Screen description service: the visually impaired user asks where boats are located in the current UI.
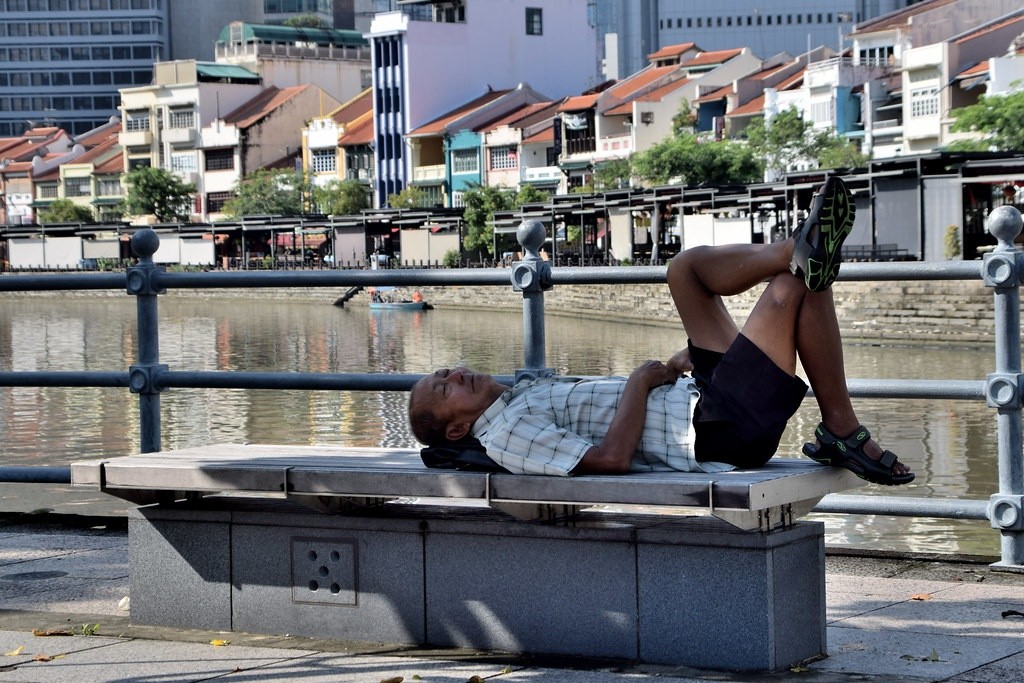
[368,299,427,310]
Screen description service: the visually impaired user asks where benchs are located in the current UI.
[71,443,868,675]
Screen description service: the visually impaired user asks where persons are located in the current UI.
[408,174,916,486]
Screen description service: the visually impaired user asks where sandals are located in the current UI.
[802,422,914,485]
[789,176,857,293]
